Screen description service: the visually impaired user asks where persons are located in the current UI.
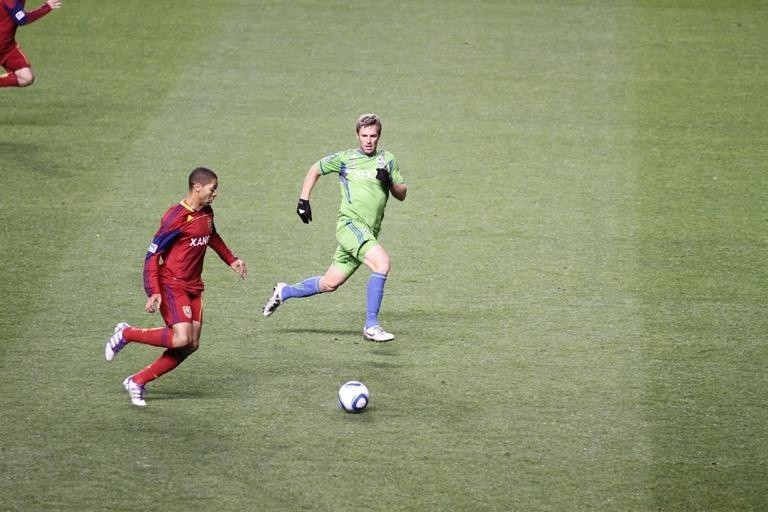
[1,0,62,87]
[262,114,408,342]
[104,167,248,408]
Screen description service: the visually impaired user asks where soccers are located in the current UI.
[339,382,369,413]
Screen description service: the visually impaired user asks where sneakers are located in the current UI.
[362,325,394,343]
[261,282,287,318]
[122,375,147,408]
[103,322,131,362]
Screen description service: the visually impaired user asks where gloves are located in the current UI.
[296,198,312,224]
[376,168,392,188]
